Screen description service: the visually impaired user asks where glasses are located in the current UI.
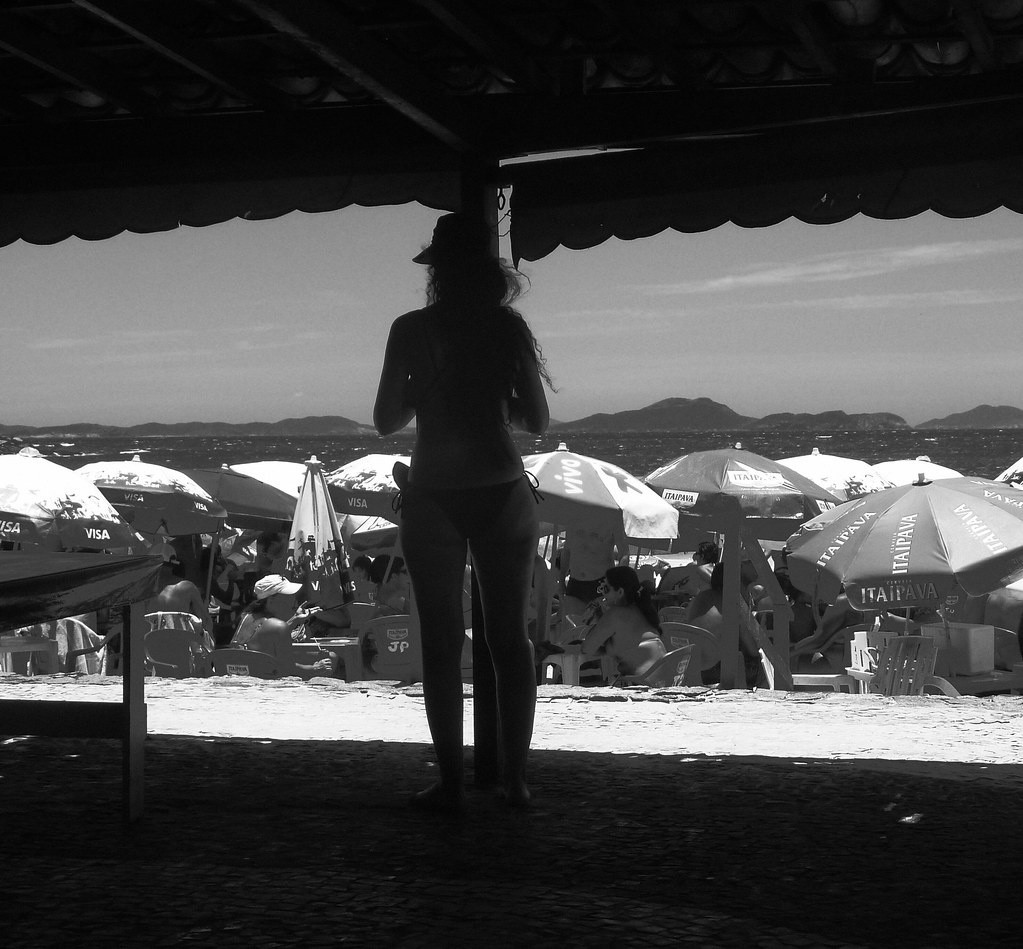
[604,586,609,594]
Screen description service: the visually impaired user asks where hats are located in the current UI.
[146,543,180,564]
[254,573,302,603]
[411,212,495,264]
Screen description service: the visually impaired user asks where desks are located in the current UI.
[792,673,856,693]
[542,644,620,688]
[291,636,363,683]
[926,670,1023,695]
[0,636,59,674]
[0,550,164,821]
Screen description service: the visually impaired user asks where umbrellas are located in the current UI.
[520,441,680,643]
[282,455,359,642]
[0,453,228,557]
[639,442,844,564]
[785,473,1023,677]
[177,461,327,612]
[773,436,1023,502]
[325,453,412,620]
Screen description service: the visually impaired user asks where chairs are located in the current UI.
[0,573,423,684]
[539,567,1023,695]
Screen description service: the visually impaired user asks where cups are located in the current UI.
[319,649,329,662]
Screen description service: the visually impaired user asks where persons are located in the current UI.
[685,542,889,689]
[373,212,551,806]
[532,503,667,687]
[144,531,411,681]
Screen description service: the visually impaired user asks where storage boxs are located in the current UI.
[920,621,995,676]
